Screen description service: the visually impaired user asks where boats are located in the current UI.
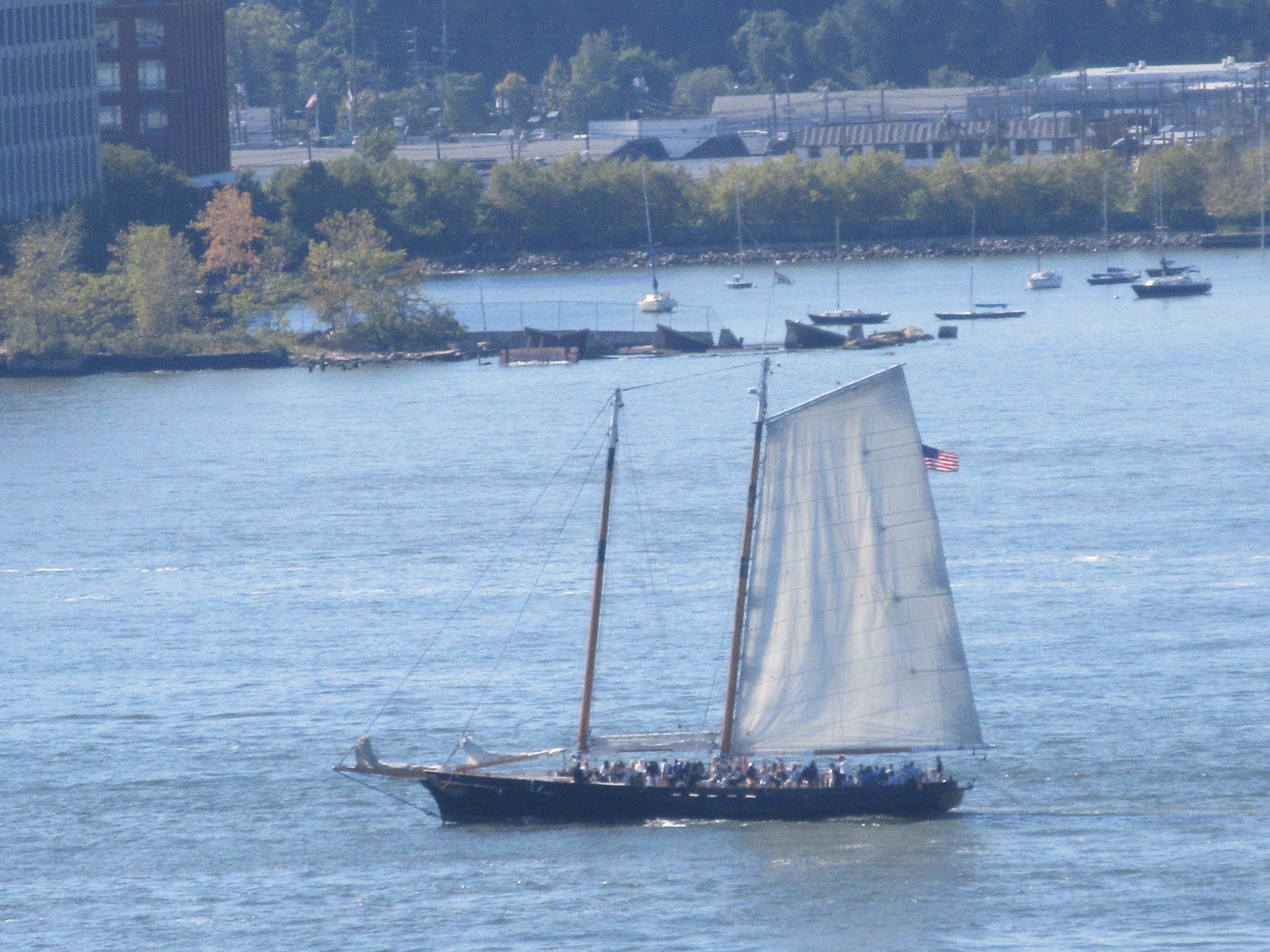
[810,310,891,325]
[1147,267,1190,276]
[637,292,677,312]
[1028,272,1061,292]
[938,310,1026,320]
[1132,272,1214,298]
[728,283,750,289]
[785,319,844,351]
[1087,272,1136,285]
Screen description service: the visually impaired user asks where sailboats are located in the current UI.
[318,366,984,824]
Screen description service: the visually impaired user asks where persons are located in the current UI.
[573,755,945,789]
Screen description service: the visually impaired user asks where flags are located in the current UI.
[924,445,960,472]
